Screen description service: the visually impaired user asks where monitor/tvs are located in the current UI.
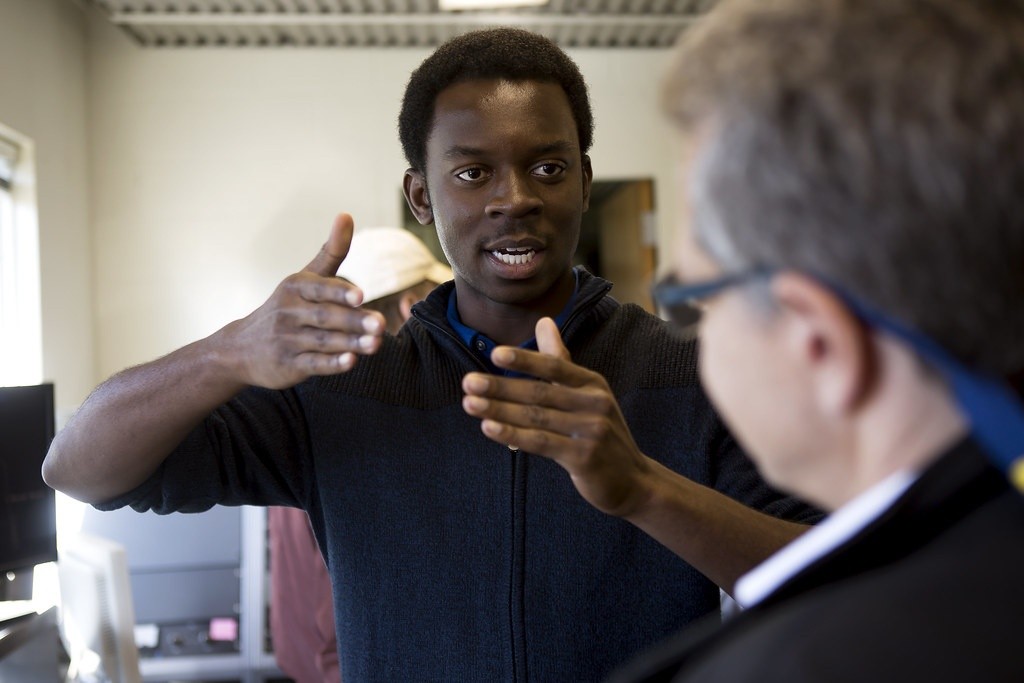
[0,381,64,625]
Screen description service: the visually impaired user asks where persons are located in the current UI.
[639,0,1024,683]
[42,28,835,683]
[268,226,456,683]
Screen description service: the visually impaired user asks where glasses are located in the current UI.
[654,260,1022,470]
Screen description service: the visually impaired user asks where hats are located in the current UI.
[335,226,454,305]
